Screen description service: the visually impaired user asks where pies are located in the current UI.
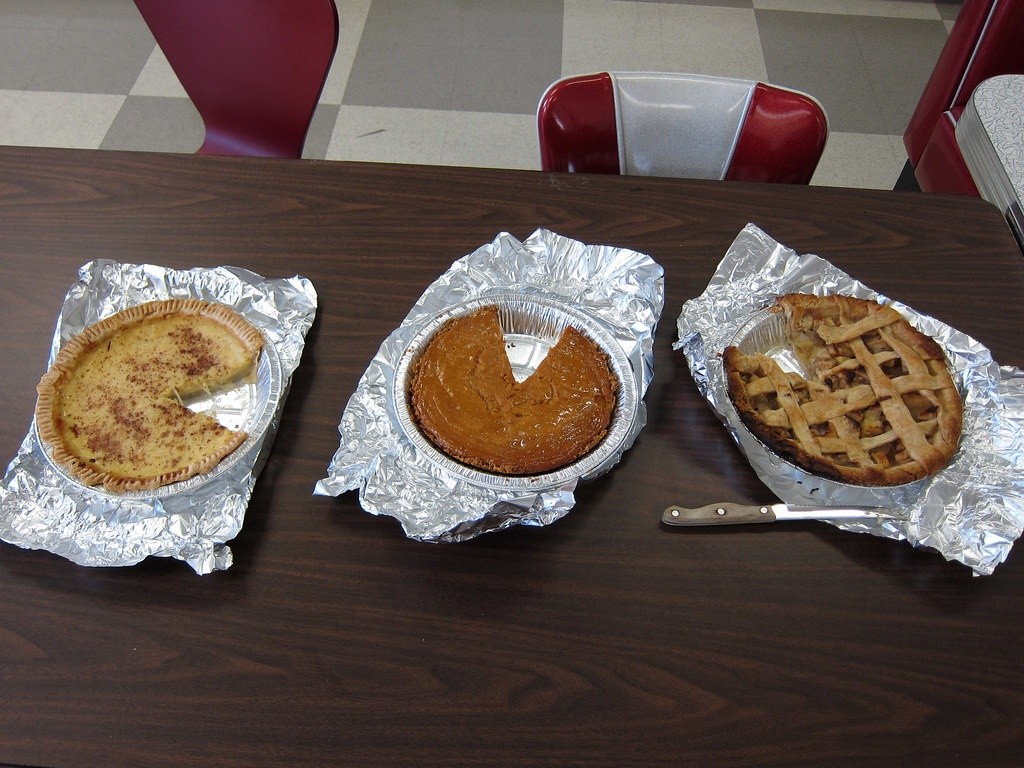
[409,305,618,477]
[722,292,964,486]
[35,299,263,491]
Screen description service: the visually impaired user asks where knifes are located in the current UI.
[661,502,908,527]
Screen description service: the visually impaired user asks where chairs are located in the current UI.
[131,0,341,161]
[536,71,832,187]
[892,0,1024,199]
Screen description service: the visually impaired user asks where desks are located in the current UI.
[0,145,1024,768]
